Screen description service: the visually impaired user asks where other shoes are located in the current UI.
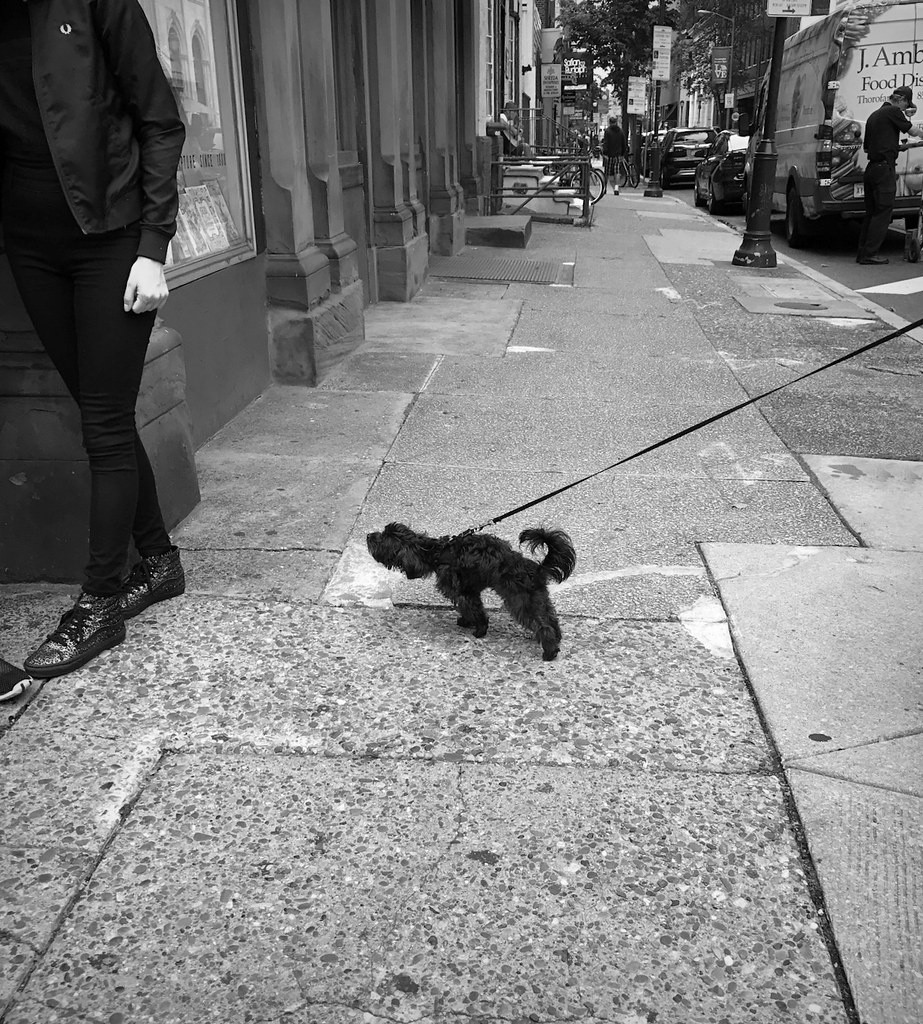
[856,256,890,264]
[614,190,619,195]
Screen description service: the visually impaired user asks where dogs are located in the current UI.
[366,522,577,662]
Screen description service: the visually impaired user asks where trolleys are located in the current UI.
[903,201,923,262]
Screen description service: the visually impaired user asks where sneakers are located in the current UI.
[23,593,127,679]
[0,659,33,700]
[119,545,186,620]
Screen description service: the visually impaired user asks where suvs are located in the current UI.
[658,126,718,190]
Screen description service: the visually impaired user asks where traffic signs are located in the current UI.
[766,0,837,18]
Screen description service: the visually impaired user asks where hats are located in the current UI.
[894,86,916,109]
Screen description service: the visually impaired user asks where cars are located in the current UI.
[692,129,750,214]
[640,128,668,180]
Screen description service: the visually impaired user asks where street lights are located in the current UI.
[678,100,684,126]
[695,93,704,127]
[697,8,736,130]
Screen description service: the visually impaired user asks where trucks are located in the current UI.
[738,0,922,249]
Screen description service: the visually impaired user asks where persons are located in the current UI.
[490,100,533,158]
[1,656,36,703]
[856,86,923,265]
[602,117,626,195]
[0,1,187,681]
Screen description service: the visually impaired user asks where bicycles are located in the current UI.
[543,148,607,206]
[613,150,640,189]
